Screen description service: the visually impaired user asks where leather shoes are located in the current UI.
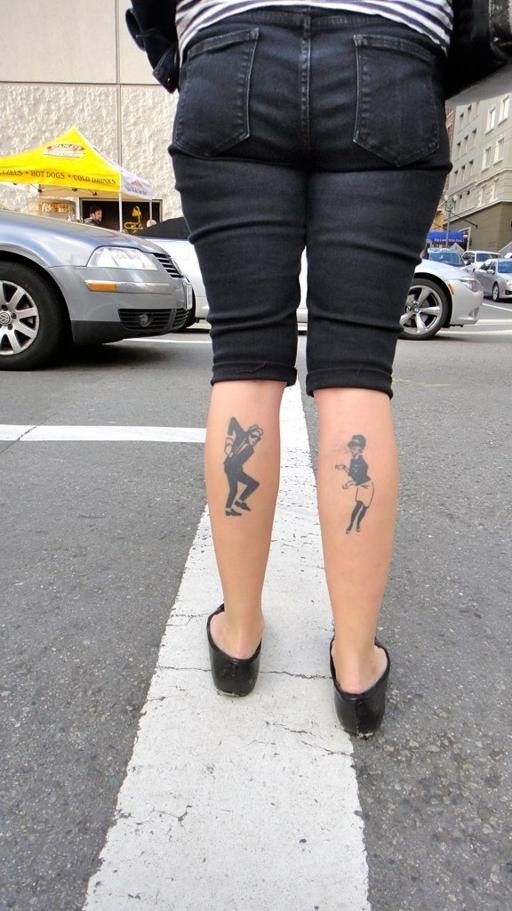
[207,603,261,696]
[330,634,389,738]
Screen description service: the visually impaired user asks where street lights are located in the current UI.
[443,194,458,247]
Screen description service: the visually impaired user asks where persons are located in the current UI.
[223,416,264,516]
[334,436,374,534]
[126,0,511,734]
[83,206,103,227]
